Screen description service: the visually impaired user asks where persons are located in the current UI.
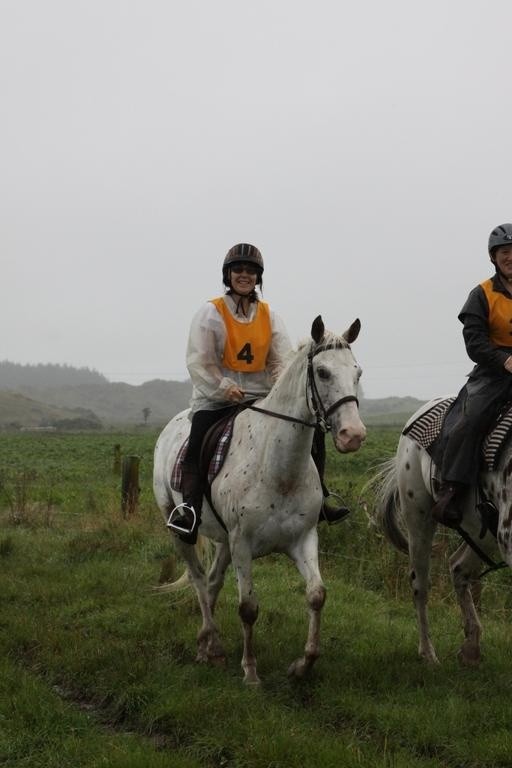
[439,219,512,527]
[172,242,350,544]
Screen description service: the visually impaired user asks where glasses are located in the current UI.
[231,265,258,274]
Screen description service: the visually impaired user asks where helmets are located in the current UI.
[223,243,264,270]
[488,223,510,252]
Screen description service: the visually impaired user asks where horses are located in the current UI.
[144,314,367,695]
[357,396,512,677]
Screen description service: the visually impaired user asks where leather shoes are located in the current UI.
[431,482,468,528]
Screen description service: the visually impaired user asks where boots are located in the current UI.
[313,430,351,524]
[168,470,202,545]
